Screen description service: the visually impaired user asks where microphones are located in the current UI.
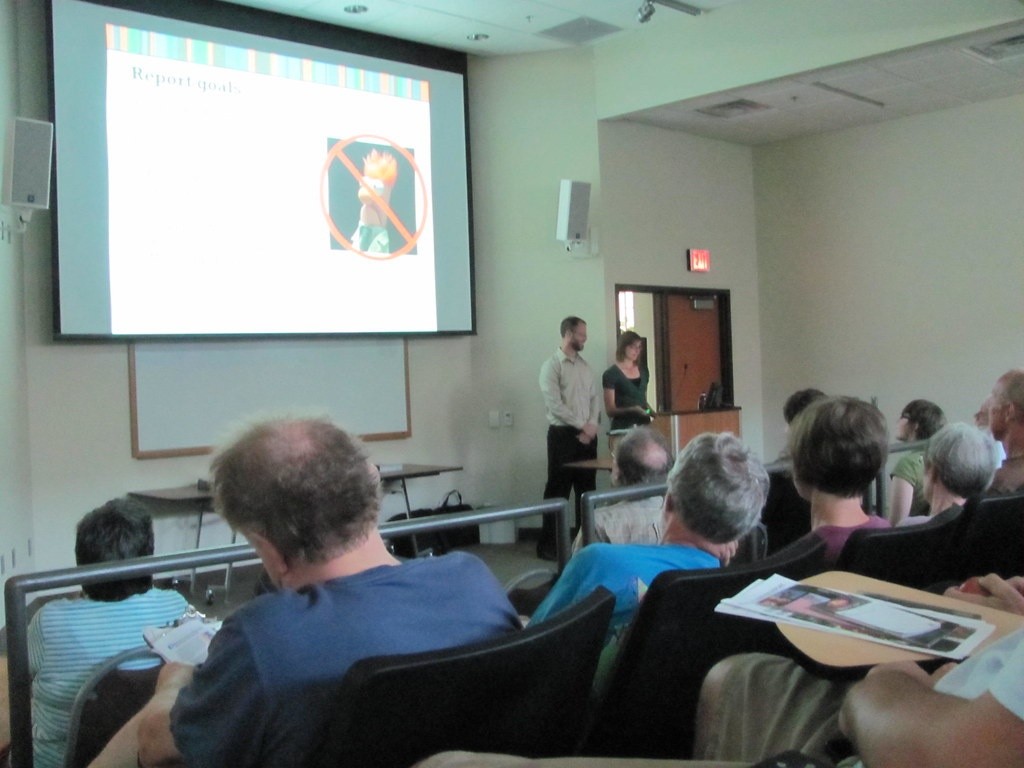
[671,364,688,411]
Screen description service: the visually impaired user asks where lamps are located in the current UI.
[635,0,656,24]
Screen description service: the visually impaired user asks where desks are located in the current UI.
[127,463,464,602]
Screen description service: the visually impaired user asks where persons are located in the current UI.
[571,430,675,554]
[520,432,770,629]
[538,316,601,560]
[783,369,1024,560]
[602,331,657,429]
[419,573,1023,768]
[89,418,527,768]
[27,497,190,768]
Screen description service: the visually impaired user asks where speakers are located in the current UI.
[5,117,53,209]
[555,179,591,242]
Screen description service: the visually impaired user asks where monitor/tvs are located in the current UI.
[704,383,723,409]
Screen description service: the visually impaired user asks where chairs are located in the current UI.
[312,583,616,767]
[63,646,165,767]
[967,484,1024,579]
[822,503,966,590]
[582,530,822,759]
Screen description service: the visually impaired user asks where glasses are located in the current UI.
[900,412,910,419]
[918,456,926,466]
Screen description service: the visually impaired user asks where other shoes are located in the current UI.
[537,549,558,562]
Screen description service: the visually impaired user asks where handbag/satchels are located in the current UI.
[385,508,447,560]
[432,489,481,551]
